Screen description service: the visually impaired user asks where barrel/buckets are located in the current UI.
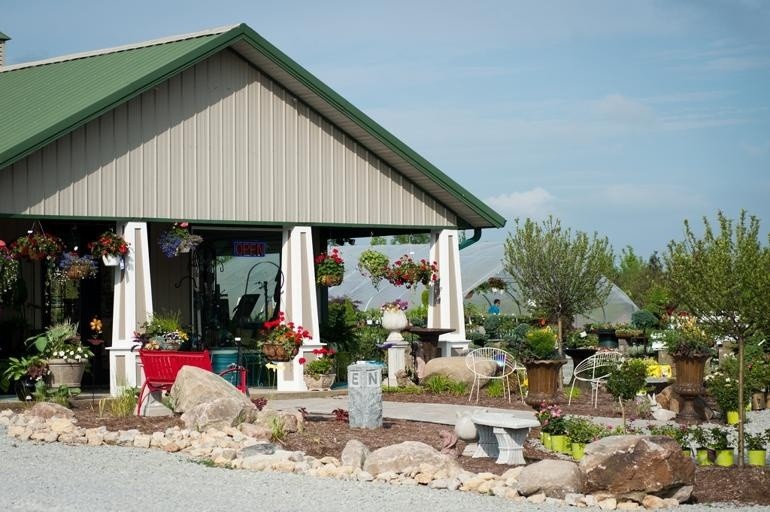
[599,330,619,349]
[210,349,243,386]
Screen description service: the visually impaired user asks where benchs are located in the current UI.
[471,408,540,466]
[137,349,247,416]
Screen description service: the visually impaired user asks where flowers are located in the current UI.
[257,311,312,357]
[0,223,130,306]
[24,318,96,363]
[299,348,337,380]
[316,249,436,291]
[130,312,190,351]
[380,298,408,312]
[157,223,203,258]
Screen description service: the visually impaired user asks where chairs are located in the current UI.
[465,347,517,404]
[568,351,625,409]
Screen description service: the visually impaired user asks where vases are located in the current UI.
[48,359,88,388]
[261,343,298,362]
[382,310,408,341]
[303,374,336,391]
[166,341,182,351]
[176,246,190,254]
[522,324,568,406]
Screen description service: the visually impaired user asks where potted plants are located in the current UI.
[660,324,766,426]
[683,427,770,467]
[540,416,604,459]
[3,356,52,402]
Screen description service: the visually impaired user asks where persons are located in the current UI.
[488,299,500,315]
